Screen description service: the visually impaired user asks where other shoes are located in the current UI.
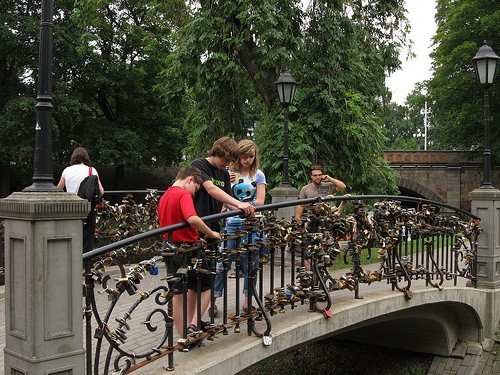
[243,305,256,312]
[187,321,210,334]
[208,305,218,317]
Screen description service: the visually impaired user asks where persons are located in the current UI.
[208,140,267,318]
[184,136,255,333]
[56,147,105,277]
[293,166,346,272]
[159,167,220,349]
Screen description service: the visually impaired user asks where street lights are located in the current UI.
[268,66,300,267]
[467,38,500,289]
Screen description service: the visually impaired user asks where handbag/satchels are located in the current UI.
[96,200,104,209]
